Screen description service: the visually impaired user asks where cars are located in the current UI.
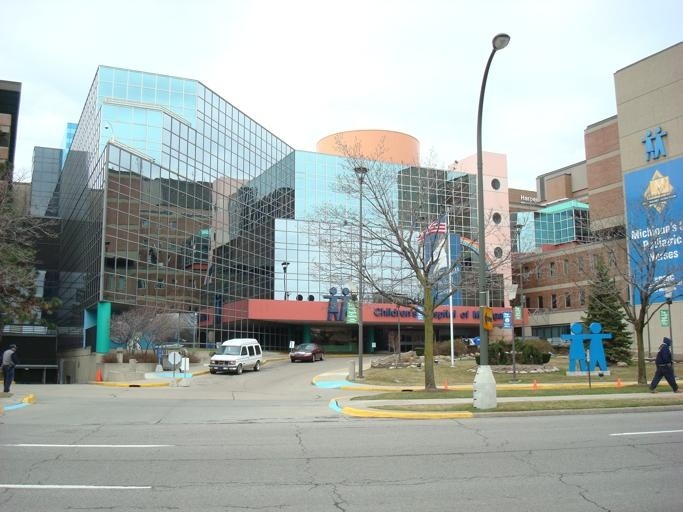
[290,343,325,362]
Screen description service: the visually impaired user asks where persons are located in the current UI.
[1,344,18,392]
[647,337,683,393]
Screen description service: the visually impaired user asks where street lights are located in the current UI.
[513,223,524,336]
[475,35,511,363]
[356,164,369,375]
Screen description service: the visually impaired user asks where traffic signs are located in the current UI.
[503,312,512,328]
[660,309,669,327]
[346,300,359,324]
[416,307,424,321]
[515,306,521,320]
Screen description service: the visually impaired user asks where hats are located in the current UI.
[7,344,20,349]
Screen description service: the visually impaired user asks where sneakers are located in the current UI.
[649,386,683,393]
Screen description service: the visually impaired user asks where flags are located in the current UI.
[415,212,447,243]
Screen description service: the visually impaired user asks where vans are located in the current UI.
[208,338,263,374]
